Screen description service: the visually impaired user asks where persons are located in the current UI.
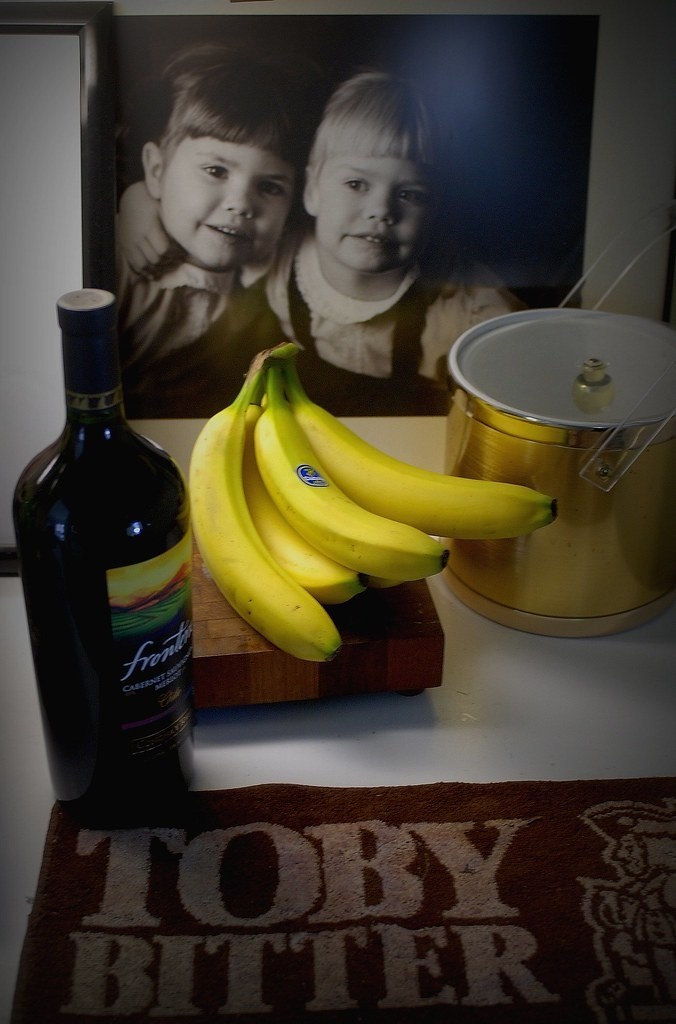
[111,47,527,421]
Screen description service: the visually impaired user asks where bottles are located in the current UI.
[15,289,198,832]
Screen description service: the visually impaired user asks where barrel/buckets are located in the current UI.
[444,193,674,636]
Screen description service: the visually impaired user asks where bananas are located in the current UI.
[186,342,559,663]
